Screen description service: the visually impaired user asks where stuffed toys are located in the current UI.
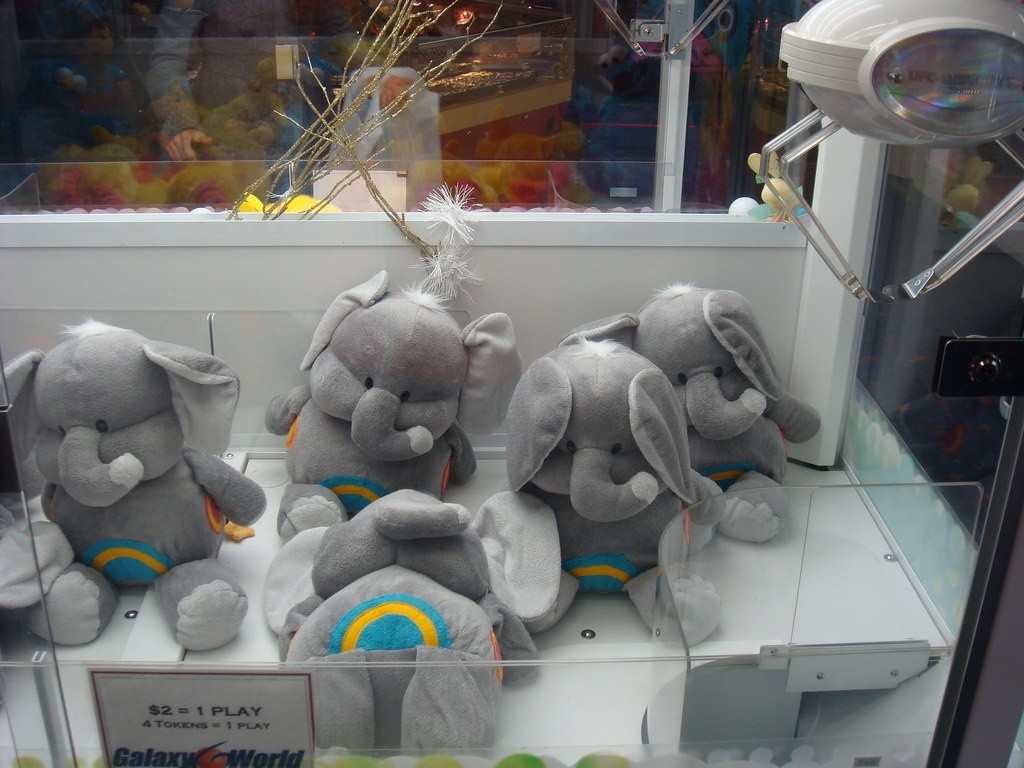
[262,487,565,760]
[34,20,323,214]
[0,312,280,651]
[263,267,525,544]
[502,280,844,651]
[382,124,596,211]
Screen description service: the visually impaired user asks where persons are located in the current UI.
[146,0,313,202]
[577,12,705,203]
